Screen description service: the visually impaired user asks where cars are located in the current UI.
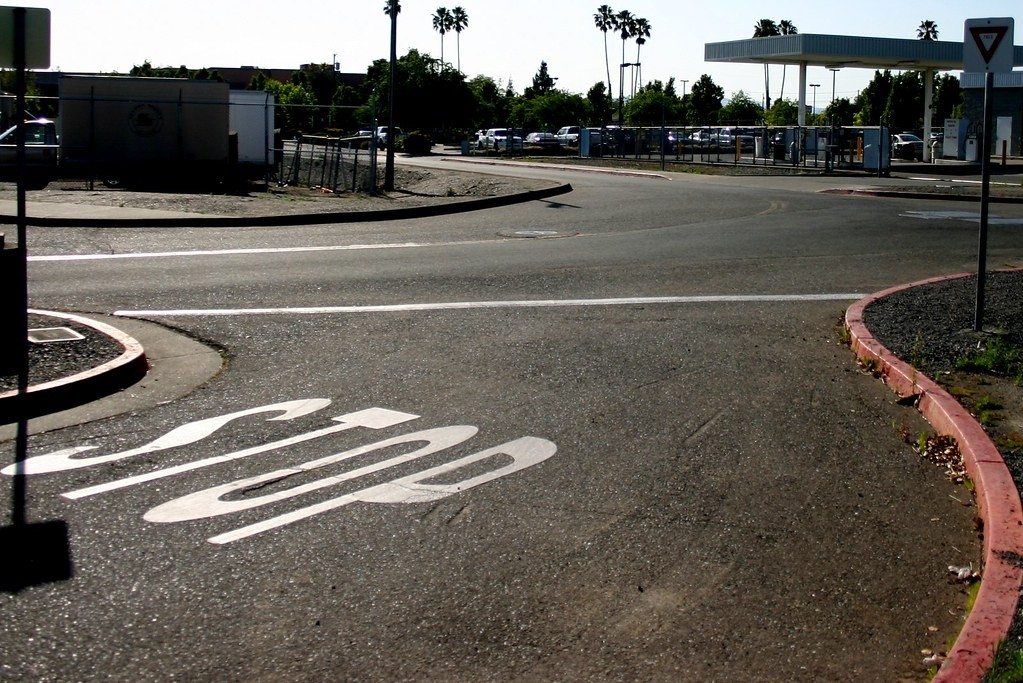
[525,133,560,146]
[930,133,940,144]
[669,132,690,142]
[864,134,924,162]
[688,127,783,147]
[354,131,372,136]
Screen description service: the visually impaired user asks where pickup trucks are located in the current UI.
[476,128,522,150]
[553,125,601,147]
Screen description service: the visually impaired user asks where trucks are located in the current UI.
[0,119,58,146]
[374,126,407,151]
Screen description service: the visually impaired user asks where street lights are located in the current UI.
[622,63,641,100]
[828,69,841,107]
[809,84,821,122]
[680,80,689,113]
